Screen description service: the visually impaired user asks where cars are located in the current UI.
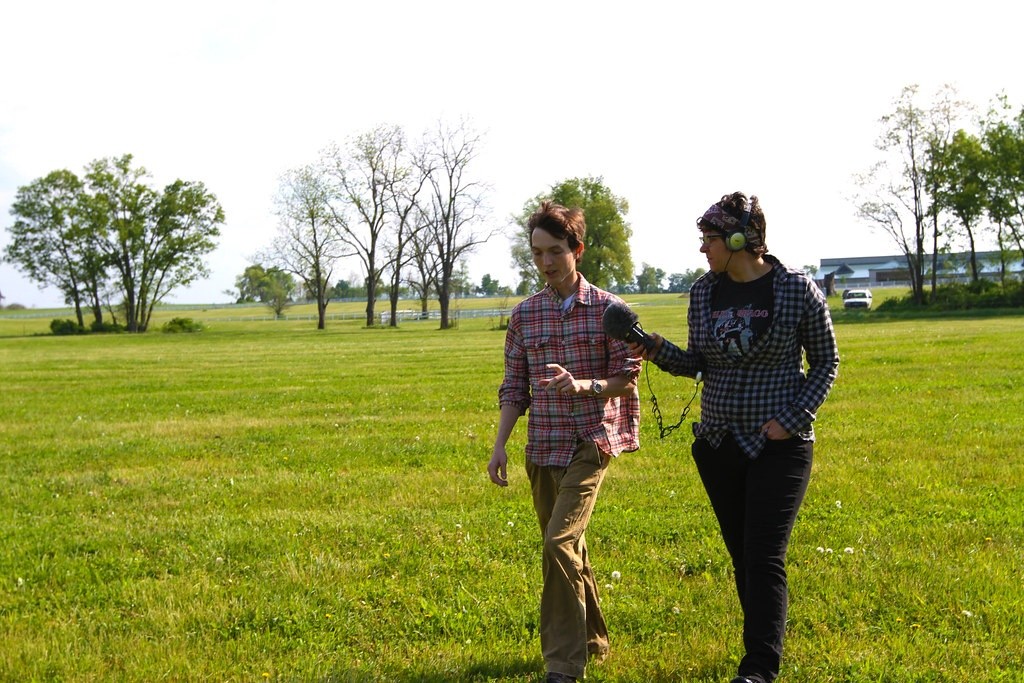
[844,289,872,310]
[841,290,851,300]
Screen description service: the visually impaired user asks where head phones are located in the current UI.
[727,199,753,252]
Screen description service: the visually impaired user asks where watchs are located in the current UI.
[588,377,604,398]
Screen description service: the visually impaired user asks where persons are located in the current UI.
[486,202,644,683]
[628,191,841,683]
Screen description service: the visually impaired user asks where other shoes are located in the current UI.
[546,671,577,683]
[730,669,773,683]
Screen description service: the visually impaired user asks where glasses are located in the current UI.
[699,234,725,245]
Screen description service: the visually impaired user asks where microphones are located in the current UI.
[600,300,658,355]
